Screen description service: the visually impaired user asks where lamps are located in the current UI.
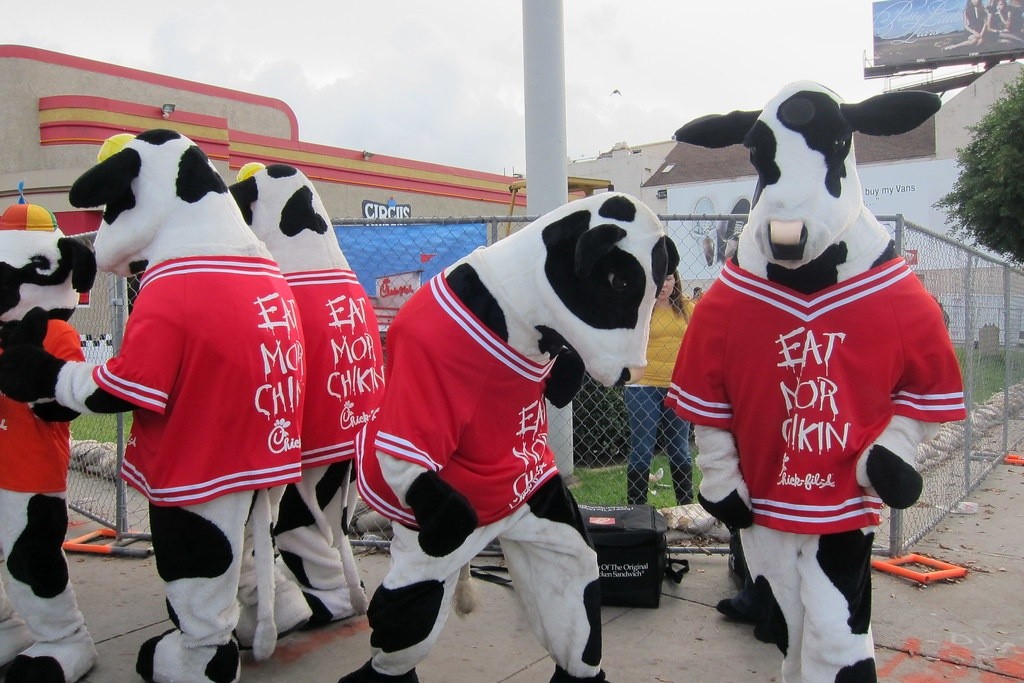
[362,150,375,157]
[657,189,667,198]
[161,103,176,114]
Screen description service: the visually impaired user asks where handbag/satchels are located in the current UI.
[575,505,689,610]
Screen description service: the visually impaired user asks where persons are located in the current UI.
[942,0,1024,50]
[626,269,695,506]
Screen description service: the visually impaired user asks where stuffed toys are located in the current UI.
[0,129,305,683]
[1,181,99,683]
[228,164,384,627]
[666,80,966,683]
[337,192,681,683]
